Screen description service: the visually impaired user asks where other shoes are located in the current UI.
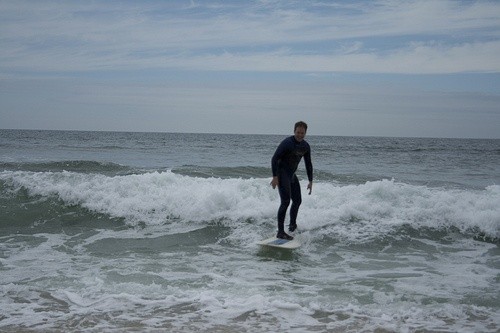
[289,223,297,231]
[277,230,293,240]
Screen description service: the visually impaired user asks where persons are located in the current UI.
[270,120,314,240]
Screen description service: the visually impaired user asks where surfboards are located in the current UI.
[254,235,302,250]
